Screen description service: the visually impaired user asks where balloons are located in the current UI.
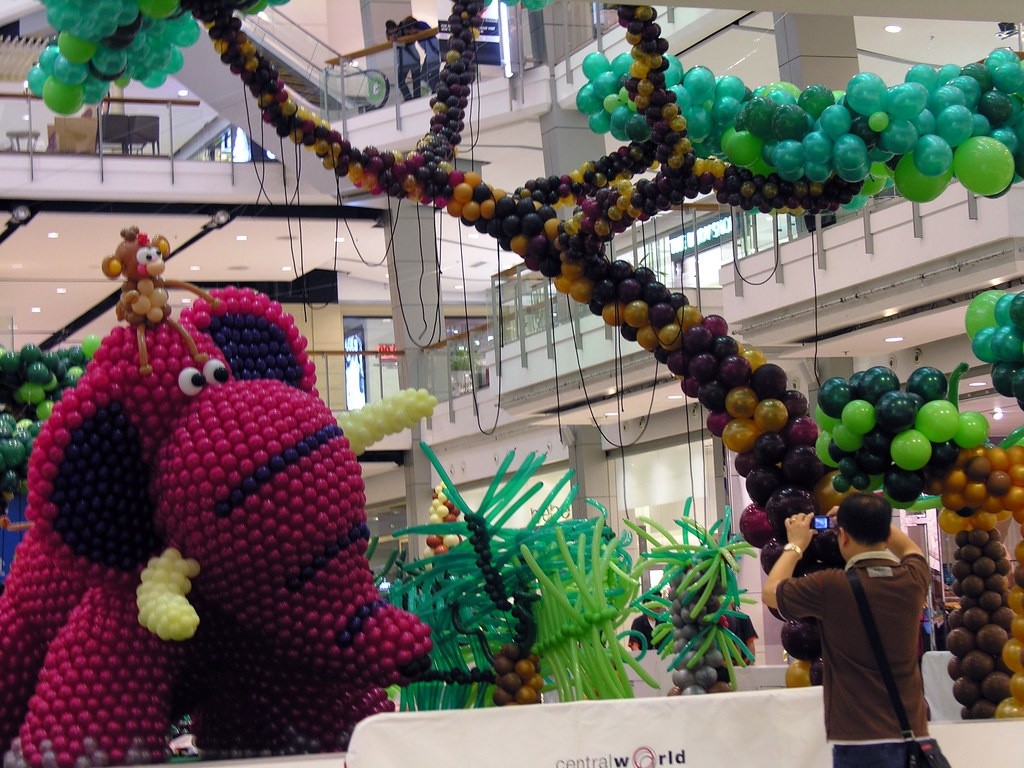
[0,0,1024,768]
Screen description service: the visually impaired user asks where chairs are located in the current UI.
[95,114,161,157]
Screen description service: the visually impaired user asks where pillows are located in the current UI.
[82,107,93,119]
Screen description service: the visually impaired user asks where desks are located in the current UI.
[6,131,40,152]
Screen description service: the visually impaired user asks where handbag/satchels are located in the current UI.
[907,739,952,768]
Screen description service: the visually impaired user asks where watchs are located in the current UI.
[784,543,802,559]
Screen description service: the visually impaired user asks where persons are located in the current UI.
[628,591,671,651]
[762,492,932,768]
[398,15,441,95]
[722,603,758,666]
[385,19,422,101]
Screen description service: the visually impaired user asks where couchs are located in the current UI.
[47,116,98,154]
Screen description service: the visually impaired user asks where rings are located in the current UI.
[790,518,796,523]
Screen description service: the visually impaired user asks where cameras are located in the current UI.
[809,516,836,531]
